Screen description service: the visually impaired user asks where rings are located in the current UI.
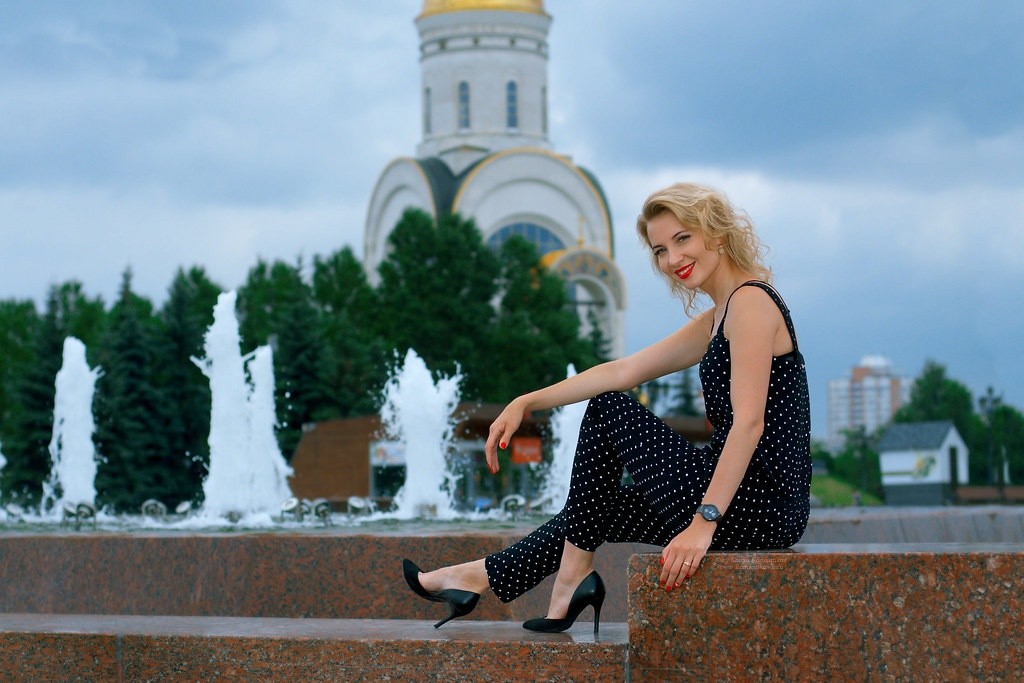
[683,562,691,567]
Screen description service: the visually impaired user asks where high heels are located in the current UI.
[523,570,606,633]
[403,559,480,628]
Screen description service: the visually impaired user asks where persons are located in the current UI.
[403,184,814,632]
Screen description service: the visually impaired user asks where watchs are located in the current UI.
[696,504,722,524]
